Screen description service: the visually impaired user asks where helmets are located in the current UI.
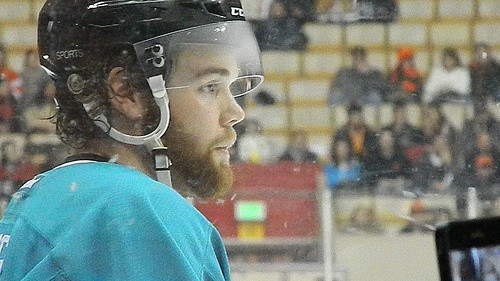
[37,0,265,188]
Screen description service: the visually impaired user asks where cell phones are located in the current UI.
[435,216,500,281]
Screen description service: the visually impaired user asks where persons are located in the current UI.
[1,0,265,281]
[1,0,500,232]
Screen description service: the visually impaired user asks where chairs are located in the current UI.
[435,219,500,281]
[0,0,500,239]
